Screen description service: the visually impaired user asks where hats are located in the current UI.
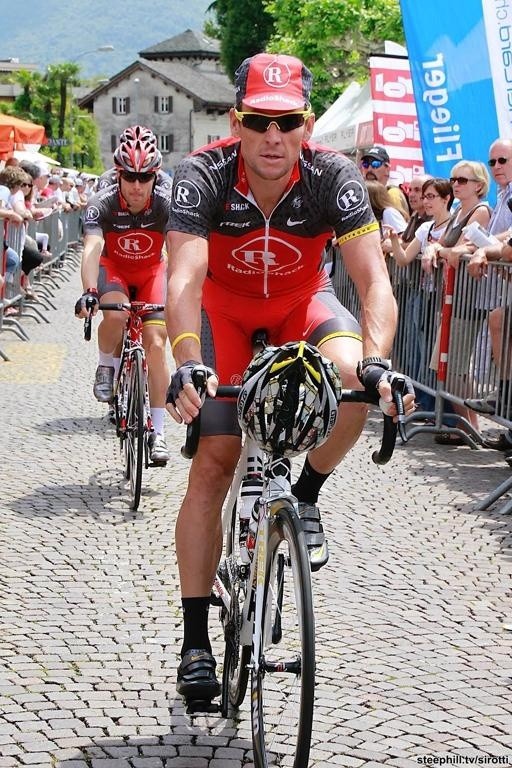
[234,53,314,112]
[360,147,390,167]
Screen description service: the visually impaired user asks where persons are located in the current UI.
[163,53,417,700]
[75,139,171,462]
[89,126,173,195]
[0,159,96,317]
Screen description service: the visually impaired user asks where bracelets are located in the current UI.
[171,332,200,357]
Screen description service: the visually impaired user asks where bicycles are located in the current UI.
[82,294,167,513]
[174,365,408,768]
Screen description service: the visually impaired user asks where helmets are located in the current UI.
[119,125,157,146]
[113,140,163,174]
[234,340,344,460]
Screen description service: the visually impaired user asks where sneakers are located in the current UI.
[176,647,222,697]
[298,500,330,568]
[434,431,484,446]
[463,388,512,416]
[93,365,115,402]
[481,434,512,451]
[147,432,170,462]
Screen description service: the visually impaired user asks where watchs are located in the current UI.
[356,356,393,386]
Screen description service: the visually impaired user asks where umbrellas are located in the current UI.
[0,113,48,153]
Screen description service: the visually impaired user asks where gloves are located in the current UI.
[362,364,416,396]
[75,293,99,315]
[165,360,219,408]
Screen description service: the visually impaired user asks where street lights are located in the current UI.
[67,45,115,168]
[74,78,111,166]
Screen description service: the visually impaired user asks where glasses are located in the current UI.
[450,177,479,185]
[363,160,388,169]
[487,157,507,167]
[235,109,312,133]
[420,193,440,200]
[22,183,33,188]
[119,171,155,184]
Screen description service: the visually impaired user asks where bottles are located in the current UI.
[239,473,269,563]
